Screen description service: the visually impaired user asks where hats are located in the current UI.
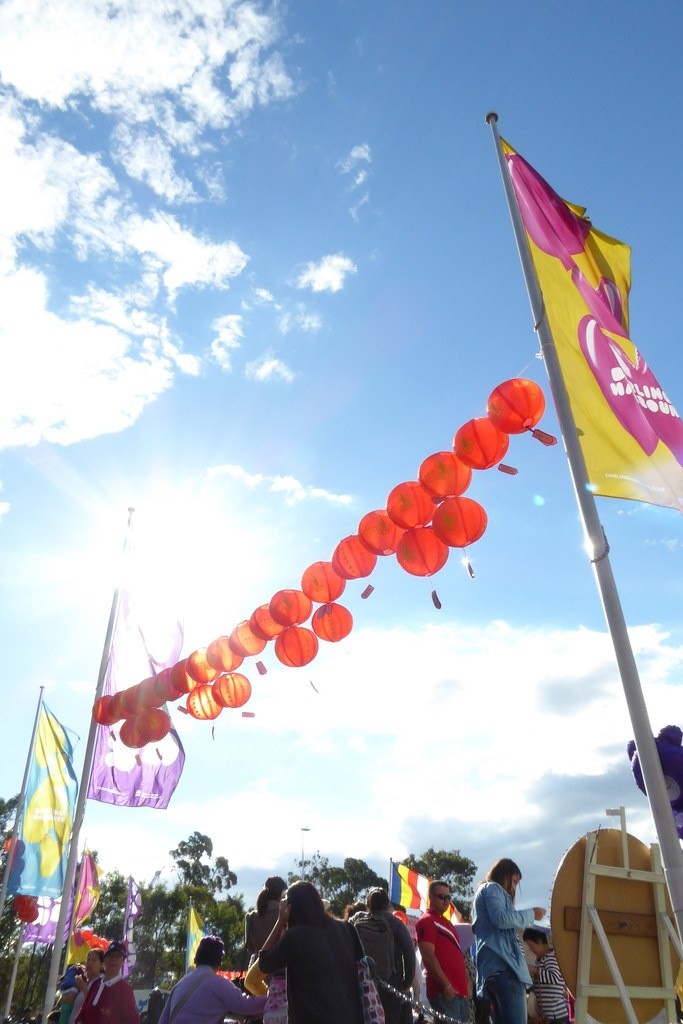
[197,936,224,959]
[265,877,287,892]
[104,940,127,960]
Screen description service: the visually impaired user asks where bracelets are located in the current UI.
[442,984,451,991]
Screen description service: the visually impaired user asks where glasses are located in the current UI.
[431,894,450,900]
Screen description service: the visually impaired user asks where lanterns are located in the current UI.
[391,910,407,926]
[92,380,545,750]
[14,894,39,923]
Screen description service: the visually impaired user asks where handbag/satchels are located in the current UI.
[228,978,250,1019]
[345,920,385,1024]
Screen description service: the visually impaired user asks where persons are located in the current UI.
[6,941,140,1024]
[159,858,570,1024]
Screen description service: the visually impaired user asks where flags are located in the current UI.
[497,136,682,513]
[87,588,185,810]
[186,904,207,973]
[389,861,465,925]
[122,875,142,981]
[22,842,76,947]
[65,850,104,966]
[7,700,80,897]
